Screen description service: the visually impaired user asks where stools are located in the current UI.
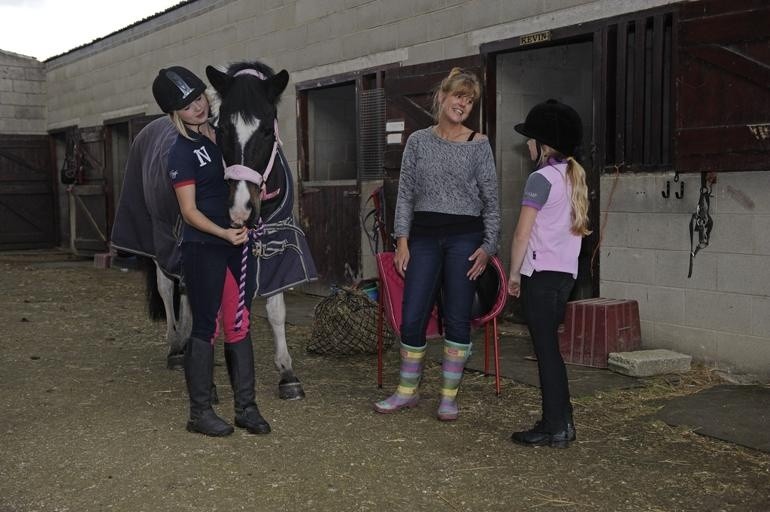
[375,252,500,396]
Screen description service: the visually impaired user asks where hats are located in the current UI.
[513,96,581,154]
[151,63,208,112]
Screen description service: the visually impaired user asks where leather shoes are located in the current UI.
[536,420,576,440]
[512,422,568,450]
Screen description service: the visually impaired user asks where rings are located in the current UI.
[480,267,483,273]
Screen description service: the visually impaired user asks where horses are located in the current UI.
[135,56,307,403]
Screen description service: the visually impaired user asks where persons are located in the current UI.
[151,66,272,436]
[507,98,595,450]
[372,66,502,421]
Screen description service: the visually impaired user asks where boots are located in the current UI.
[225,331,272,435]
[373,339,429,413]
[183,339,234,438]
[437,336,473,421]
